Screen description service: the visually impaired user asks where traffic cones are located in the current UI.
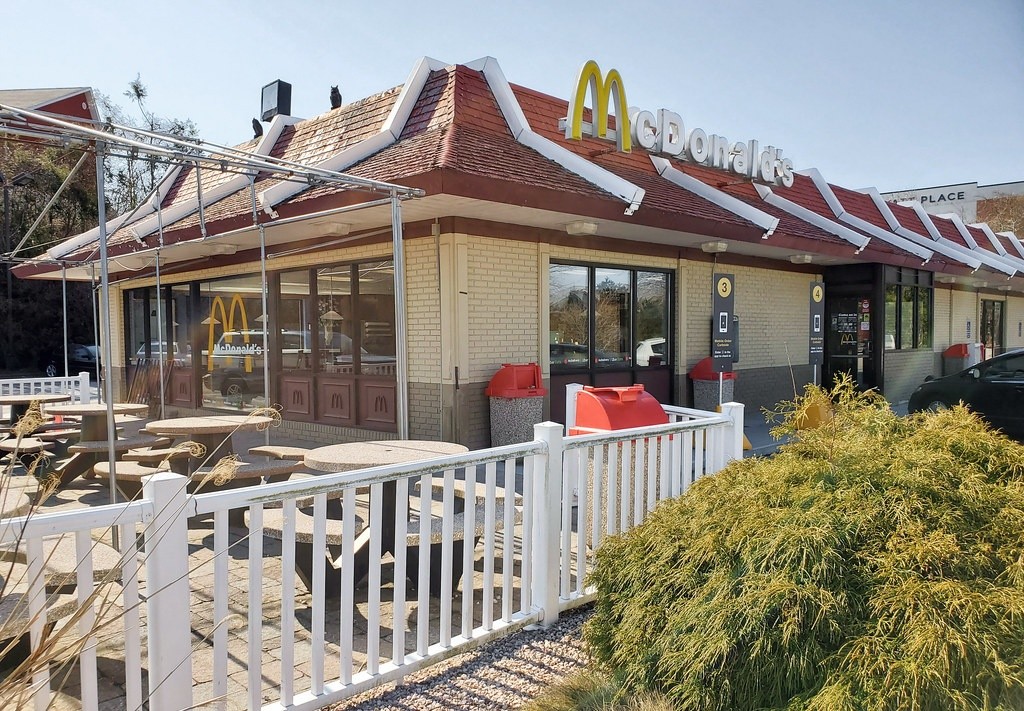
[52,414,67,433]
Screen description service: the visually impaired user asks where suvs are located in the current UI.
[202,328,369,407]
[135,342,208,372]
[637,337,667,367]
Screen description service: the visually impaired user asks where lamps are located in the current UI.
[565,221,597,237]
[790,255,813,264]
[141,258,165,267]
[319,222,351,237]
[701,240,728,253]
[210,244,238,255]
[86,269,101,276]
[201,282,221,324]
[253,275,268,322]
[319,269,344,321]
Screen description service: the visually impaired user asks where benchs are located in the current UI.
[58,436,170,489]
[94,460,168,502]
[64,416,82,430]
[248,446,309,484]
[121,447,192,476]
[0,416,55,428]
[0,427,46,459]
[190,461,306,525]
[243,486,370,601]
[32,426,124,460]
[0,437,57,495]
[407,477,523,598]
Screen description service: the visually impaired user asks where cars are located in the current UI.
[40,342,102,377]
[907,349,1024,441]
[550,343,629,368]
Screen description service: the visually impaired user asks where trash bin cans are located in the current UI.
[943,343,971,377]
[484,360,548,464]
[688,354,737,420]
[569,383,675,550]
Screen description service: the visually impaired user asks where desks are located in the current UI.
[0,394,72,438]
[303,439,470,552]
[145,415,273,522]
[43,403,150,478]
